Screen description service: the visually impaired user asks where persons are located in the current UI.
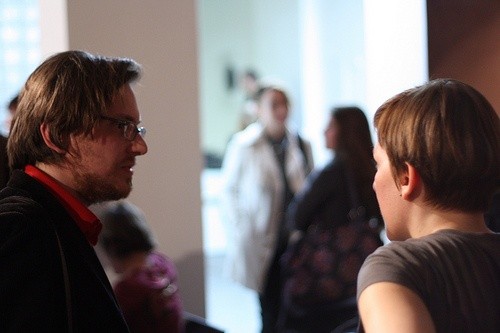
[260,105,384,332]
[357,77,500,333]
[220,85,314,295]
[0,48,148,333]
[95,198,183,332]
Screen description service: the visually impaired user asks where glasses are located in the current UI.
[93,114,146,141]
